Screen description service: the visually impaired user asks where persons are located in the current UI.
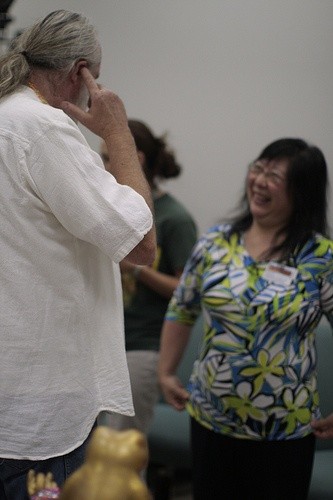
[159,137,332,499]
[98,118,198,352]
[0,9,158,499]
